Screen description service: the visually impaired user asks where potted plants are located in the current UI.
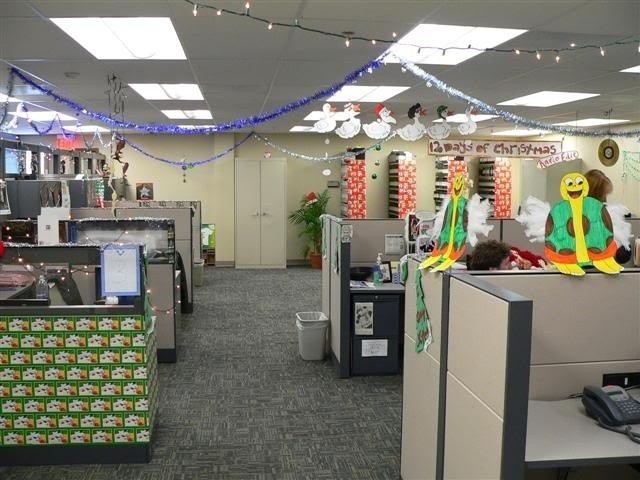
[287,189,332,269]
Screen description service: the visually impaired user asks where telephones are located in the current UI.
[582,386,640,444]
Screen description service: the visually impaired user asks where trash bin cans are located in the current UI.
[295,312,330,361]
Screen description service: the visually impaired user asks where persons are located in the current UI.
[584,169,632,264]
[469,240,514,270]
